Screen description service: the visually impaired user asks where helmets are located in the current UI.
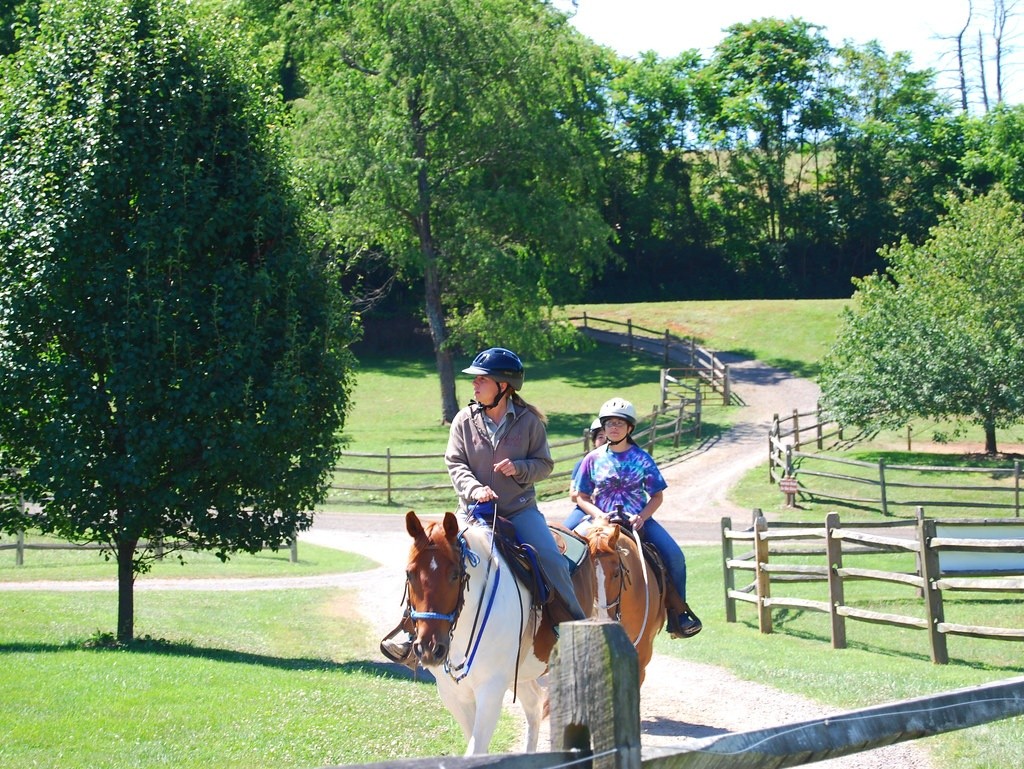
[599,398,636,426]
[590,419,602,431]
[461,348,524,390]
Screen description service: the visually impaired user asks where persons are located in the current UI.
[379,346,583,663]
[562,418,606,532]
[572,397,704,638]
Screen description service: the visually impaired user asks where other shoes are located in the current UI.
[671,621,700,640]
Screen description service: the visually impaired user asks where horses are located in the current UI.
[404,510,668,758]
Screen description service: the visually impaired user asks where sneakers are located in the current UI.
[382,640,421,671]
